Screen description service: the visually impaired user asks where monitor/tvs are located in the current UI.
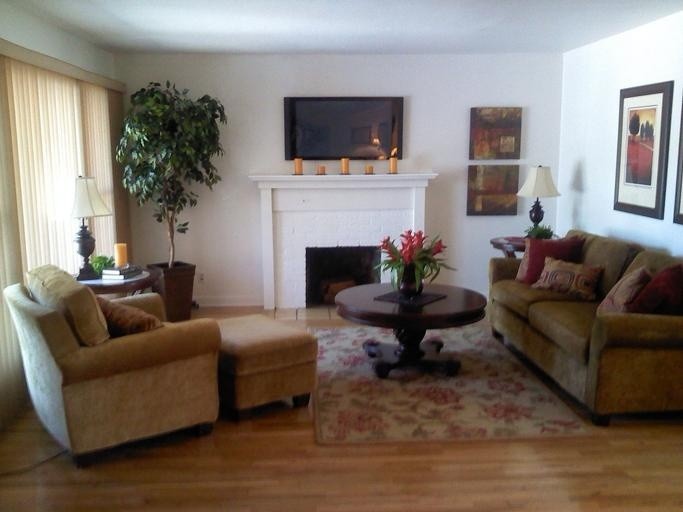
[284,97,404,160]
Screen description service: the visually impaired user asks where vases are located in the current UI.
[396,270,425,302]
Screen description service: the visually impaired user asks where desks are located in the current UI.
[78,269,156,299]
[334,282,488,379]
[490,235,553,261]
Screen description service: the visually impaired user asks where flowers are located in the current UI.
[377,229,459,302]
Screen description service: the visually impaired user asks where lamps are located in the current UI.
[515,163,561,239]
[69,172,114,281]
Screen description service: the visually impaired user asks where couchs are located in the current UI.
[3,261,222,471]
[217,312,320,423]
[485,228,682,429]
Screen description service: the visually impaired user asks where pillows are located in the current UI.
[626,265,682,315]
[596,266,653,314]
[95,292,165,338]
[531,255,606,308]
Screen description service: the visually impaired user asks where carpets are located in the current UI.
[310,324,596,446]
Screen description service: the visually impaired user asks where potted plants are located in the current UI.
[116,80,228,325]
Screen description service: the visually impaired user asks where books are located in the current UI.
[102,264,141,274]
[100,269,142,280]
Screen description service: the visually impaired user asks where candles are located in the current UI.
[113,242,129,272]
[292,147,401,177]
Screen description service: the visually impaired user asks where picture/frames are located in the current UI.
[671,86,683,226]
[612,79,675,220]
